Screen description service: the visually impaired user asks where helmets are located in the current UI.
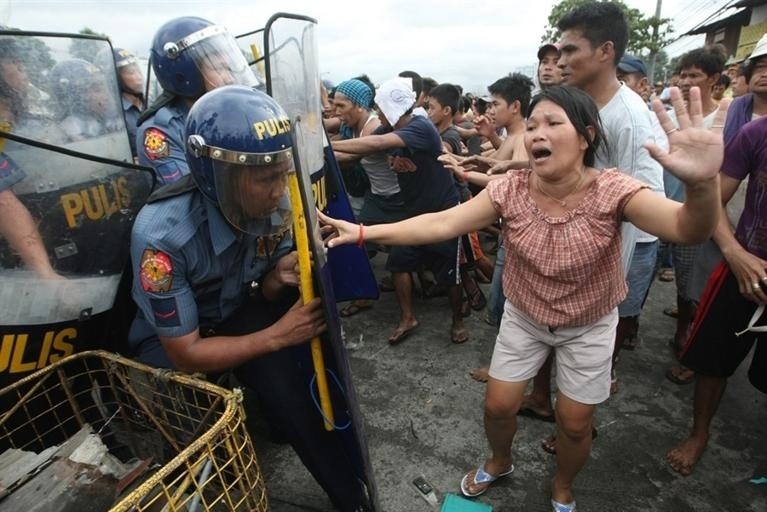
[184,85,295,237]
[49,16,260,97]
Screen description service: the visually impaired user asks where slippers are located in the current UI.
[666,363,695,384]
[516,407,555,422]
[461,461,514,497]
[550,498,576,512]
[542,427,597,454]
[390,318,419,344]
[664,308,679,318]
[659,270,674,282]
[421,276,487,342]
[338,299,373,317]
[622,331,637,350]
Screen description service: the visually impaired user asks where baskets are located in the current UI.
[1,349,271,512]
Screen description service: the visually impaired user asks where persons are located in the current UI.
[0,1,766,512]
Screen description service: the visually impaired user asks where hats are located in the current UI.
[537,43,561,61]
[618,55,647,76]
[748,33,767,59]
